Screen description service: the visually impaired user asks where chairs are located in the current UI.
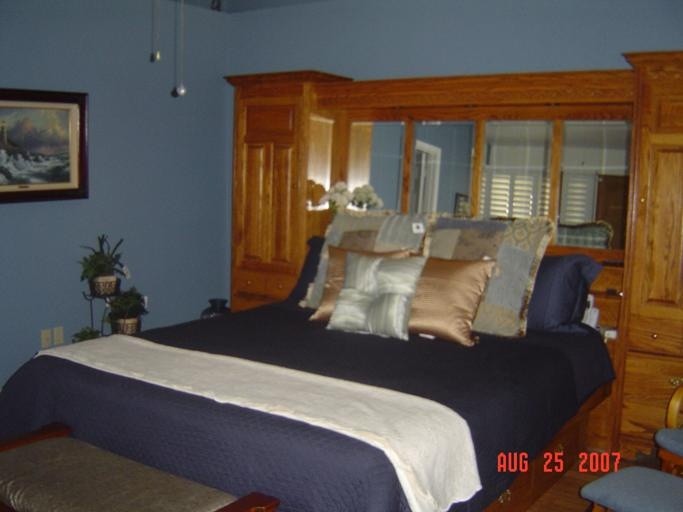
[580,386,683,512]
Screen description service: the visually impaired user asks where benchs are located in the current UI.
[2,424,282,512]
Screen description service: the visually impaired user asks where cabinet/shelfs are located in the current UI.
[613,63,683,460]
[221,69,354,310]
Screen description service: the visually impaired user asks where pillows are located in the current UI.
[422,214,556,338]
[326,253,425,343]
[409,258,496,346]
[300,211,434,309]
[309,244,415,321]
[525,255,600,334]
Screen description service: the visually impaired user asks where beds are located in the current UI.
[1,234,624,512]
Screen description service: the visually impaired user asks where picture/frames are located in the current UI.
[1,86,90,203]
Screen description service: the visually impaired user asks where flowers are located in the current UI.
[317,180,354,209]
[352,183,385,209]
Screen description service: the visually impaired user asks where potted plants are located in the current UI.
[75,233,150,335]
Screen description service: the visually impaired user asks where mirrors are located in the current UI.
[337,102,632,253]
